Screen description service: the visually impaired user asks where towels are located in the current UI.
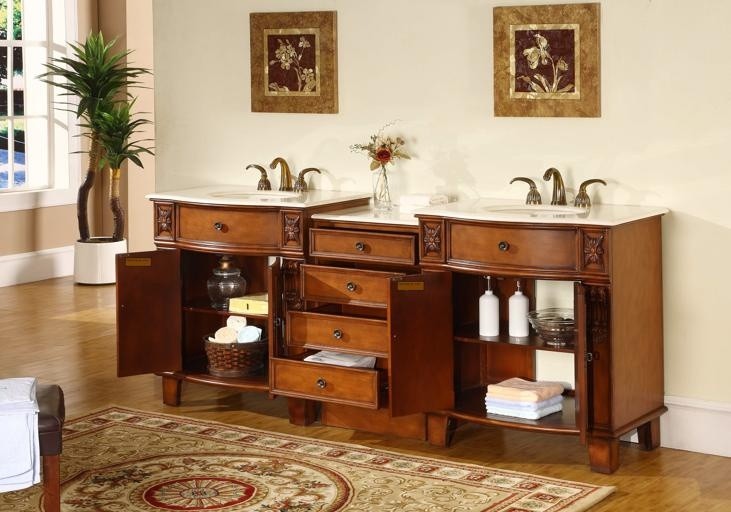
[0,377,41,494]
[207,314,263,345]
[481,375,567,420]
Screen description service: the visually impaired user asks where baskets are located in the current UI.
[202,334,263,378]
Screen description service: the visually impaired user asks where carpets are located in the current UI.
[0,403,619,512]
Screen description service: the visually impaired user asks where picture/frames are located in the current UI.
[245,10,345,117]
[490,1,607,120]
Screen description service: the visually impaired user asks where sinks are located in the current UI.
[482,204,590,216]
[205,191,299,205]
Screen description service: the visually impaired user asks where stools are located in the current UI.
[0,374,67,512]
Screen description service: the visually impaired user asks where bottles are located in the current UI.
[206,256,248,309]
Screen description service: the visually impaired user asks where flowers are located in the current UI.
[266,34,319,92]
[350,120,414,202]
[515,29,577,96]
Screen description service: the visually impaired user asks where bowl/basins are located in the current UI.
[526,308,576,348]
[210,315,262,342]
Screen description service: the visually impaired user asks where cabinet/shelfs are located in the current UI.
[385,210,671,475]
[112,180,370,428]
[267,198,451,448]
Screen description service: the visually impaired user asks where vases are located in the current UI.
[368,170,398,219]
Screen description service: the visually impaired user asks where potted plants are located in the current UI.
[36,29,152,285]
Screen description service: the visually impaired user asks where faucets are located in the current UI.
[543,168,567,205]
[270,157,294,191]
